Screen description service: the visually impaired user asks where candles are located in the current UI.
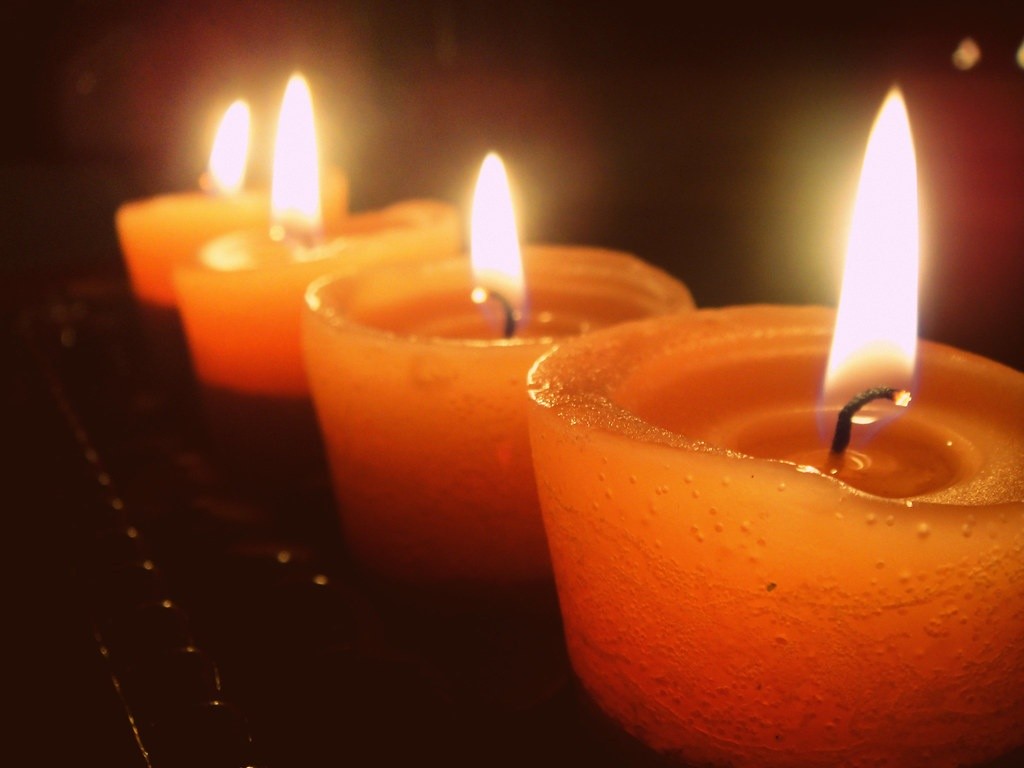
[173,72,466,414]
[113,96,353,313]
[298,147,701,595]
[520,84,1024,768]
